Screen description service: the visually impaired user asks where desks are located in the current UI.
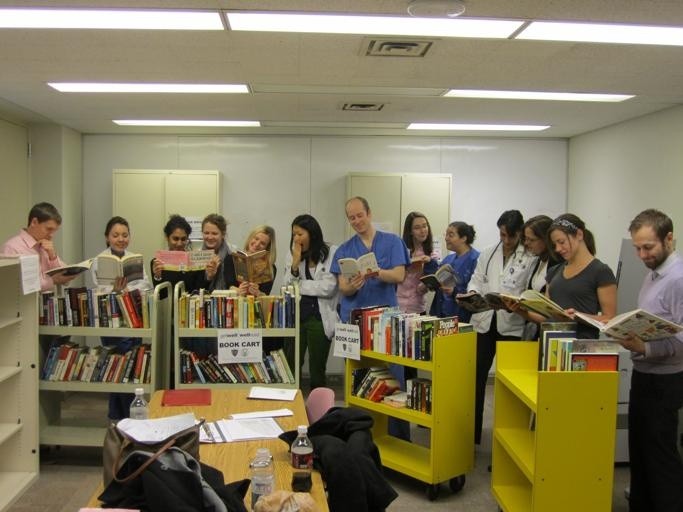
[88,389,329,512]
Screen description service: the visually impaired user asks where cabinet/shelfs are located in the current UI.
[174,281,300,389]
[111,169,220,287]
[346,173,452,266]
[39,280,172,448]
[345,331,477,499]
[491,340,619,512]
[1,256,39,511]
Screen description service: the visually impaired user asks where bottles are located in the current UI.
[290,425,313,492]
[250,447,275,508]
[129,388,150,420]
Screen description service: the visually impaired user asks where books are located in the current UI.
[352,366,432,415]
[181,287,296,330]
[39,286,152,328]
[536,321,622,371]
[351,304,459,362]
[180,347,295,383]
[42,343,152,383]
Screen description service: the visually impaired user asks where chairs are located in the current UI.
[304,386,334,425]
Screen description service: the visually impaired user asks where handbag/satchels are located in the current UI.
[103,418,205,487]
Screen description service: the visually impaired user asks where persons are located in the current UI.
[328,194,683,512]
[283,213,338,391]
[4,202,278,426]
